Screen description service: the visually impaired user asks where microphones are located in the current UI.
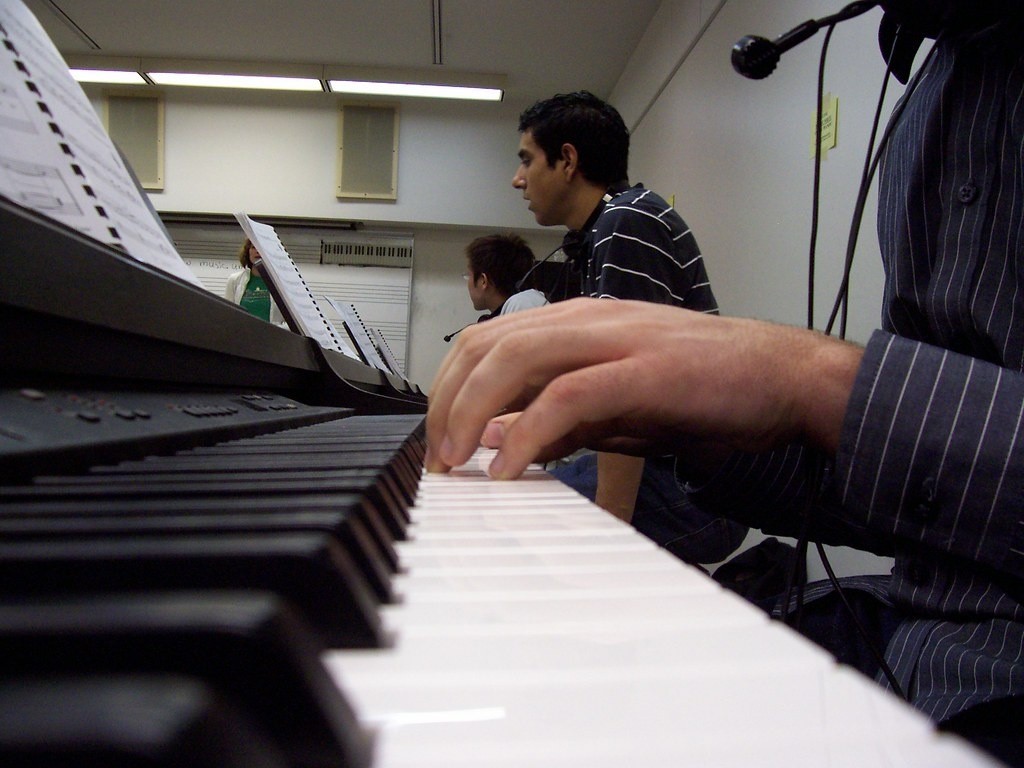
[444,323,476,342]
[517,242,570,292]
[731,0,880,80]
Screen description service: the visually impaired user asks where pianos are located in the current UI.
[0,192,1015,768]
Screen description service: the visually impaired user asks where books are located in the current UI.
[0,1,410,381]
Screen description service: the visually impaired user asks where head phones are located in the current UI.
[561,180,631,259]
[478,304,505,323]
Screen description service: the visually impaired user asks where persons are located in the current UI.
[228,242,285,328]
[414,1,1024,768]
[462,91,753,567]
